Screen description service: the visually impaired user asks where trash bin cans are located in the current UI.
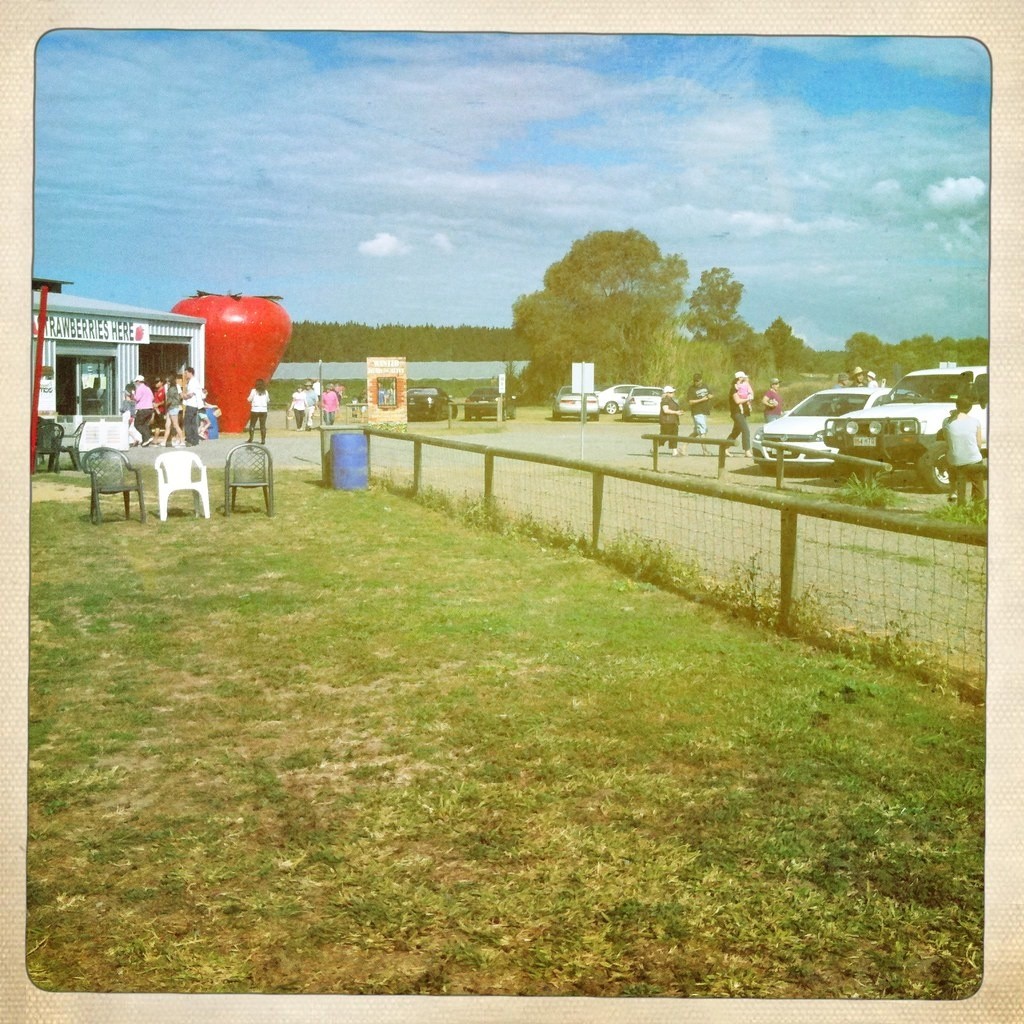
[331,434,369,489]
[205,407,219,440]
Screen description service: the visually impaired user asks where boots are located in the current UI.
[261,429,266,444]
[247,427,254,442]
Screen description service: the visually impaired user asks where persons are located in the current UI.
[677,373,714,457]
[833,367,878,388]
[247,378,270,444]
[650,386,686,456]
[942,396,983,500]
[351,396,359,411]
[378,380,395,405]
[762,378,783,423]
[120,366,218,449]
[725,371,753,458]
[288,380,343,431]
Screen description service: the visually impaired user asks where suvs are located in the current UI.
[824,364,989,493]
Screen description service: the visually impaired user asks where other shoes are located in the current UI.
[679,448,689,456]
[141,437,153,446]
[745,451,753,458]
[297,427,302,432]
[673,449,680,456]
[725,449,734,457]
[305,427,311,431]
[700,451,713,456]
[155,444,166,449]
[198,432,206,440]
[175,444,186,447]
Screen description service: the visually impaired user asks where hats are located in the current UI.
[735,371,747,378]
[134,375,145,382]
[853,367,866,376]
[867,370,876,377]
[770,378,781,385]
[304,382,313,386]
[693,373,702,380]
[838,373,848,381]
[663,386,676,393]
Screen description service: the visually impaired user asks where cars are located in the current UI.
[592,383,646,415]
[551,385,600,422]
[621,385,664,422]
[465,386,517,420]
[750,387,919,475]
[405,387,458,421]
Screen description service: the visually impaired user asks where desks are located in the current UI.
[346,403,368,424]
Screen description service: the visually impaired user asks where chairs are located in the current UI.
[32,421,64,474]
[55,420,86,474]
[80,447,147,526]
[154,451,211,521]
[816,402,832,416]
[223,443,274,517]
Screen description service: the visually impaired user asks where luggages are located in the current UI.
[286,410,296,430]
